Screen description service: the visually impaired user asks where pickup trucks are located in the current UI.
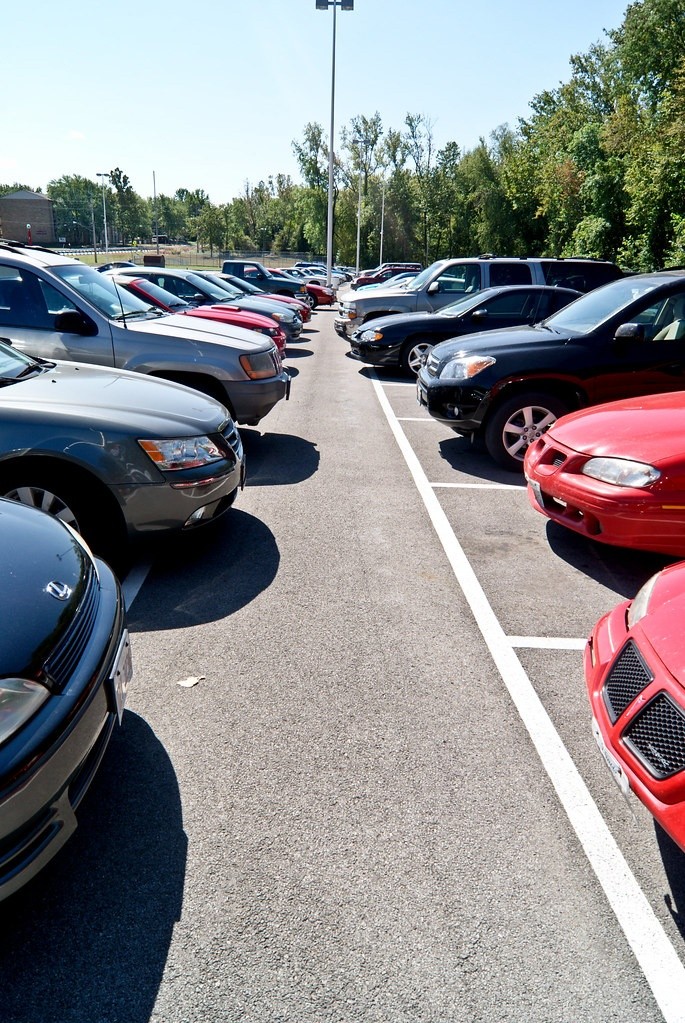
[221,261,308,301]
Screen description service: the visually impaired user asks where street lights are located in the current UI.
[96,173,110,250]
[315,0,354,287]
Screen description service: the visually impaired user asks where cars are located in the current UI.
[360,272,466,296]
[102,276,286,356]
[585,558,685,857]
[94,261,136,273]
[244,268,334,310]
[0,336,247,551]
[524,390,685,558]
[415,266,685,471]
[0,496,133,903]
[351,284,585,378]
[278,261,357,291]
[100,267,304,342]
[188,270,317,323]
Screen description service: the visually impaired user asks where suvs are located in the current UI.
[351,268,420,291]
[0,235,291,428]
[359,263,422,279]
[334,254,624,342]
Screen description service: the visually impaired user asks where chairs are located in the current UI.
[164,280,178,296]
[653,299,685,340]
[465,276,480,292]
